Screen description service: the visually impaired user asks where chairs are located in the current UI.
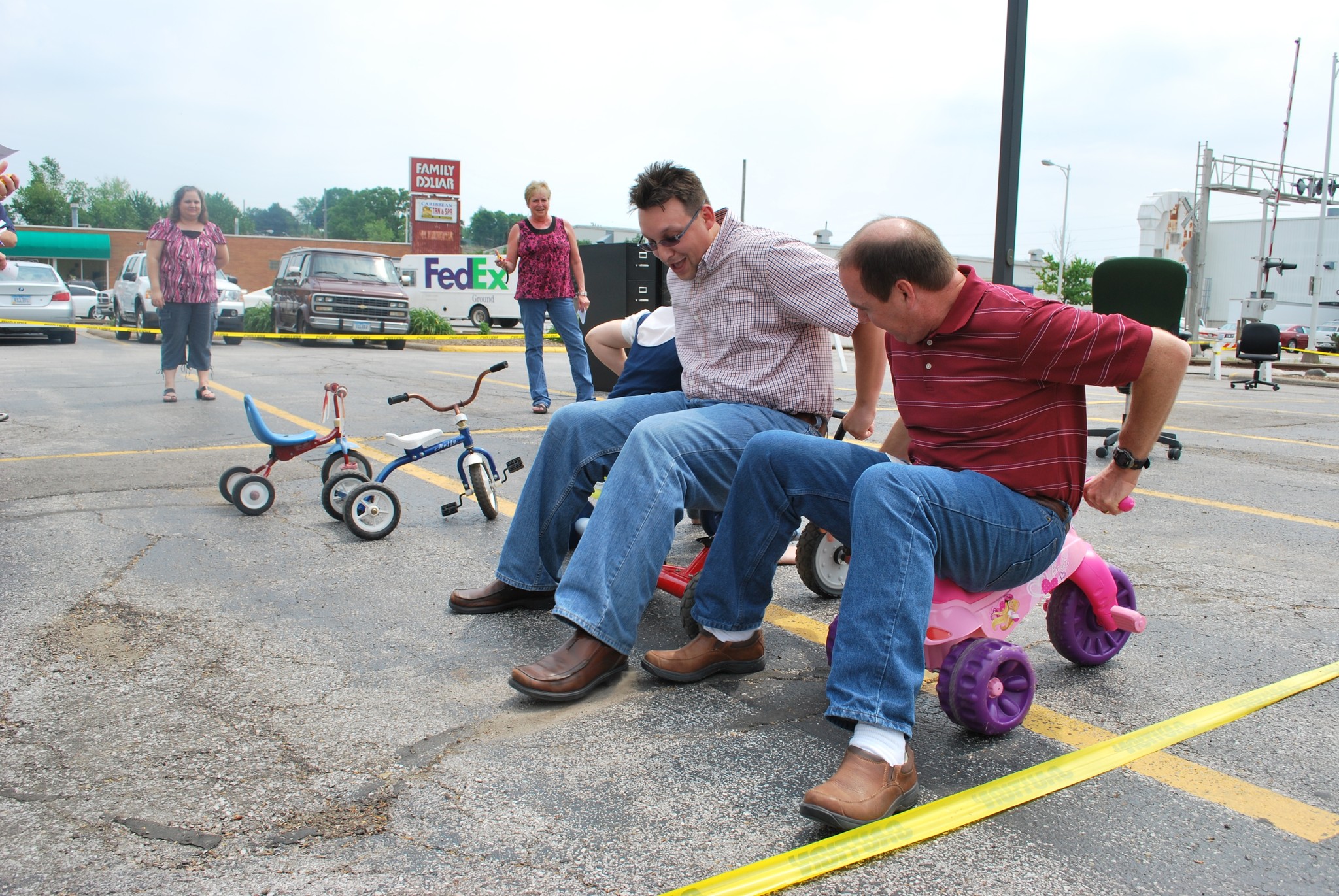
[1231,323,1281,391]
[1087,256,1187,460]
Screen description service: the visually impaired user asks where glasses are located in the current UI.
[637,200,706,252]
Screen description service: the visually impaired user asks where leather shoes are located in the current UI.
[800,746,919,832]
[642,630,768,682]
[507,627,629,700]
[449,578,558,614]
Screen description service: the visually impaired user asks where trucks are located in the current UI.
[396,254,552,328]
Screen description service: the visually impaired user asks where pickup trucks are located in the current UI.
[1178,317,1219,351]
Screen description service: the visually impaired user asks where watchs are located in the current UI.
[577,292,587,296]
[1112,446,1150,470]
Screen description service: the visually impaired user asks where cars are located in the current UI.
[242,286,275,311]
[1218,322,1240,343]
[64,279,115,321]
[0,260,76,344]
[1275,324,1311,354]
[1309,319,1339,352]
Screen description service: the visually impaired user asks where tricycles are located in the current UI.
[217,359,527,541]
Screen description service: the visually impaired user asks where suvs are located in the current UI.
[273,247,412,351]
[112,250,245,345]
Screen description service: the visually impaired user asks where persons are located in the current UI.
[493,180,599,413]
[145,186,230,402]
[448,161,889,702]
[641,216,1191,830]
[0,160,20,422]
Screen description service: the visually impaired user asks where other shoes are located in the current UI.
[0,413,9,422]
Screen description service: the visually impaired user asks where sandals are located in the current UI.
[163,388,177,402]
[196,386,216,400]
[533,403,547,413]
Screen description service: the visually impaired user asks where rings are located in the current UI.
[587,306,589,308]
[1101,511,1109,514]
[855,437,861,440]
[498,261,500,264]
[0,195,6,198]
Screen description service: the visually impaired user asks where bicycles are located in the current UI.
[826,482,1137,735]
[577,408,851,601]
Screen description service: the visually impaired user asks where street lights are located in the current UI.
[1042,161,1072,301]
